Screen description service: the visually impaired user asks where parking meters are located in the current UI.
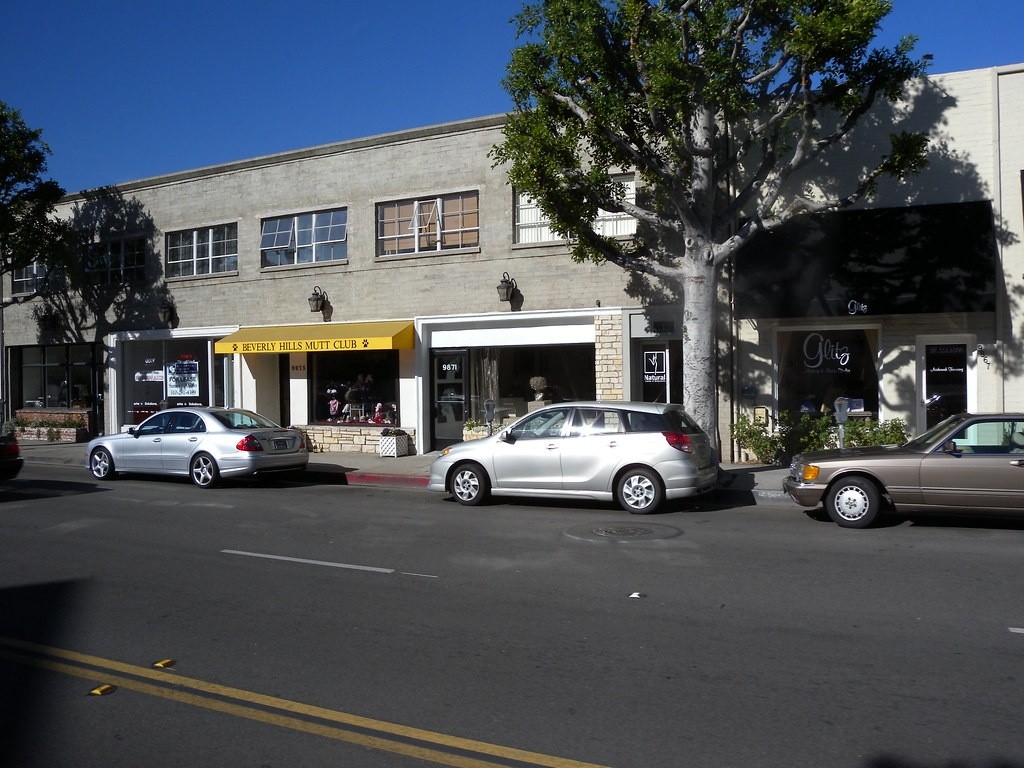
[834,397,850,448]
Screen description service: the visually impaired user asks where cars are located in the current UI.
[84,406,309,489]
[781,413,1024,528]
[428,401,718,515]
[0,436,23,480]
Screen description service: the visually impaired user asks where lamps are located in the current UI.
[157,298,173,324]
[308,286,325,313]
[496,271,514,302]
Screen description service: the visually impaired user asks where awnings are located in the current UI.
[214,321,414,353]
[733,199,997,320]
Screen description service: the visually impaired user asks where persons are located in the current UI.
[327,394,338,421]
[341,401,350,423]
[375,400,382,422]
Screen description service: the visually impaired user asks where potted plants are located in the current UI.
[379,428,409,458]
[3,413,88,443]
[462,418,491,441]
